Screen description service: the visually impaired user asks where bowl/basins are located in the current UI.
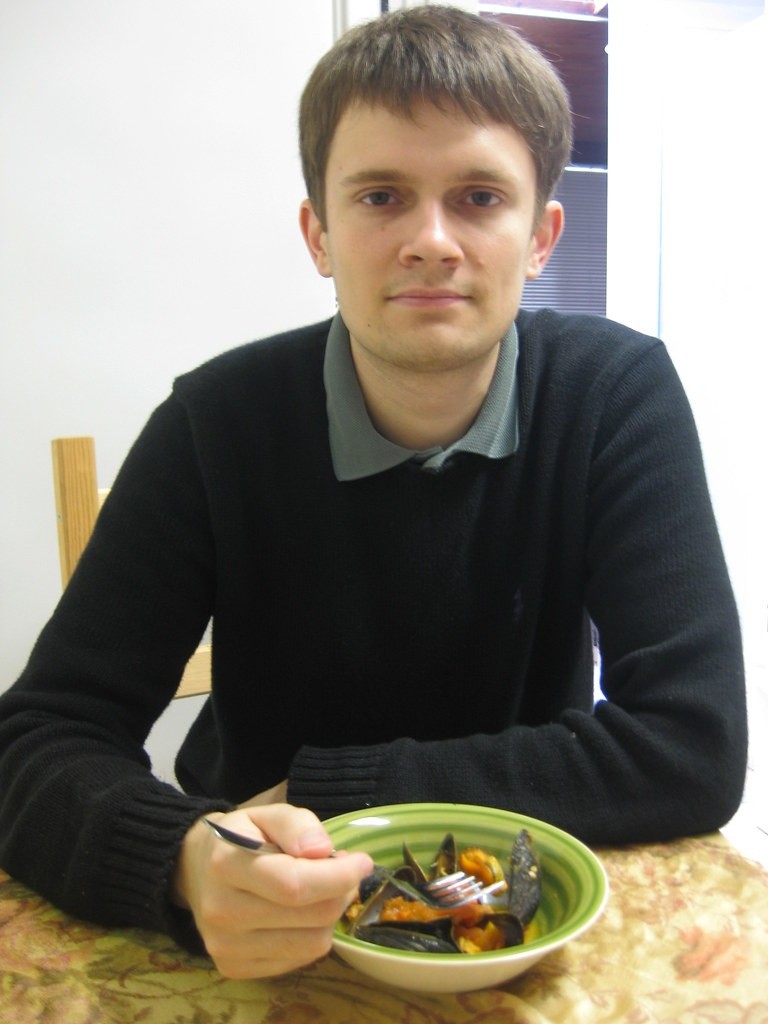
[322,802,609,992]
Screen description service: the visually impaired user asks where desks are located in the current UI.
[0,830,767,1024]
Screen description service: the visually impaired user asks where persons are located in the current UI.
[1,6,749,980]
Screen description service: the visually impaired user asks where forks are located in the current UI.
[202,817,505,910]
